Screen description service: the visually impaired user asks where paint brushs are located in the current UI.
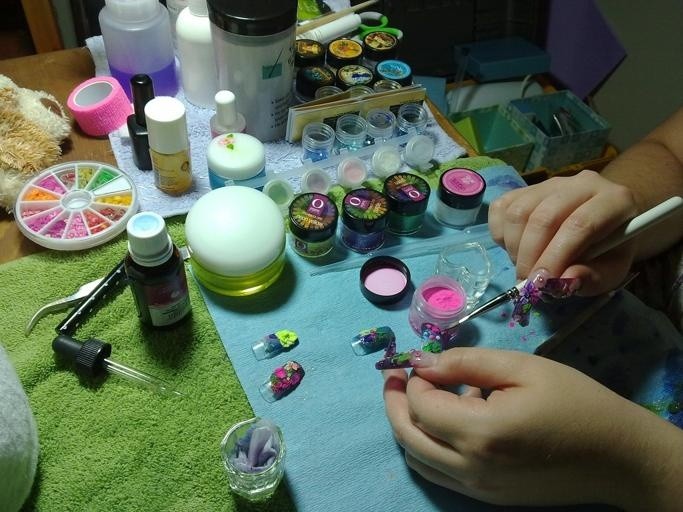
[445,195,683,331]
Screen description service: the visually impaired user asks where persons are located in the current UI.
[384,108,683,512]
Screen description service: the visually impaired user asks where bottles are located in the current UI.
[125,210,191,329]
[142,90,247,197]
[97,0,179,104]
[167,0,217,107]
[206,0,297,142]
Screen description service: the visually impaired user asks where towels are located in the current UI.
[89,38,468,215]
[2,158,497,510]
[189,164,683,510]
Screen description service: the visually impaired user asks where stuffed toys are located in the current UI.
[0,74,71,214]
